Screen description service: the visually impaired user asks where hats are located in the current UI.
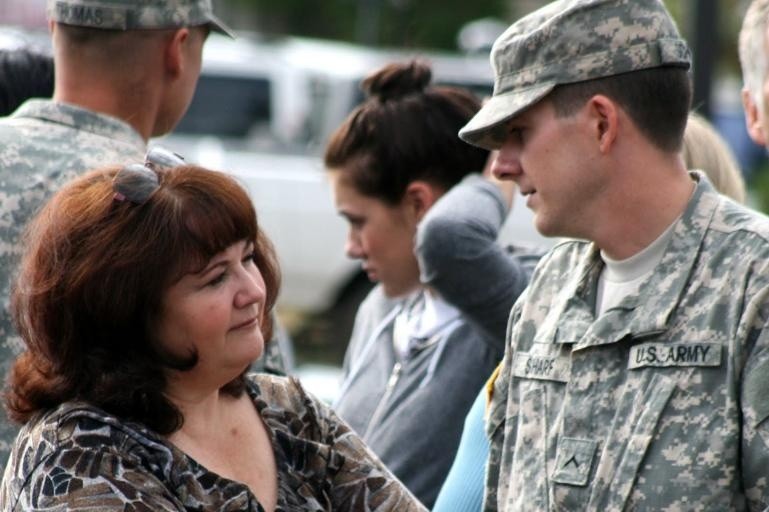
[458,1,692,150]
[49,0,238,41]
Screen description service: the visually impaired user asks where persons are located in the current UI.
[323,1,769,512]
[0,1,434,512]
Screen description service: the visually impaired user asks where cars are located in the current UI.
[159,34,569,365]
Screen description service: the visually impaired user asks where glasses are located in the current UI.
[109,145,188,214]
